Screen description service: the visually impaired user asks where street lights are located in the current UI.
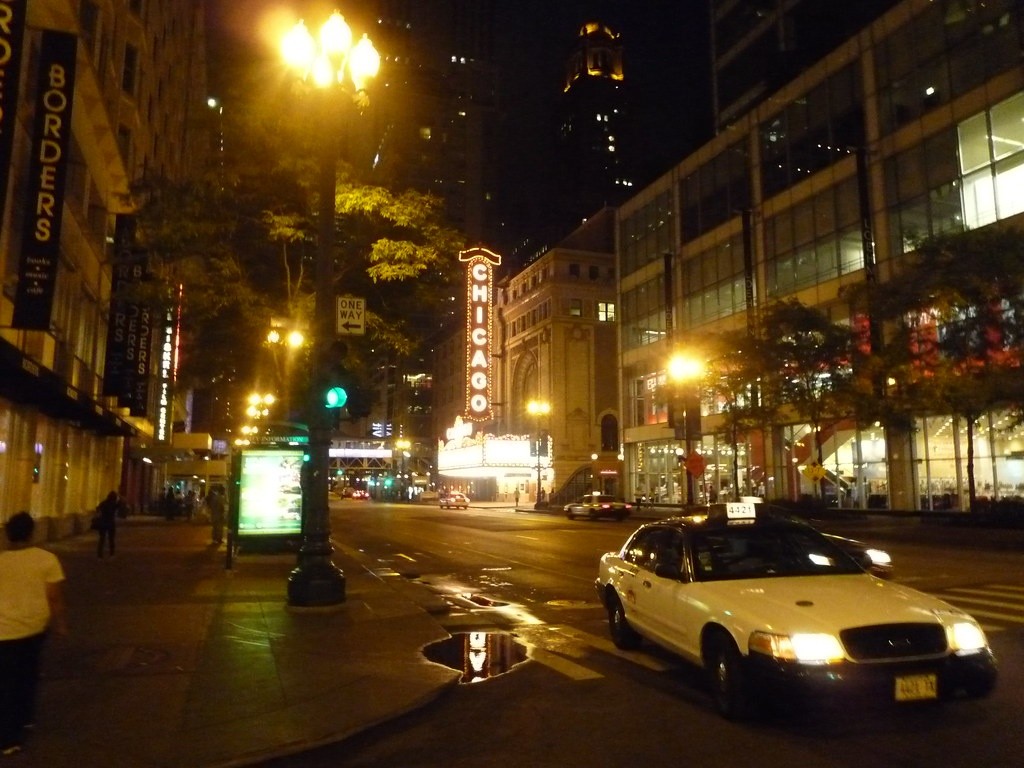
[279,5,380,608]
[526,402,551,503]
[667,347,705,515]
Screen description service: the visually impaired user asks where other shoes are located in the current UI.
[22,718,40,729]
[3,740,24,755]
[213,538,224,544]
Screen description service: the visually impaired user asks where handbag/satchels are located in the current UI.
[88,516,100,530]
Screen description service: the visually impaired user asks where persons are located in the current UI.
[634,485,717,511]
[844,475,871,509]
[0,511,68,752]
[97,491,120,559]
[341,487,346,500]
[513,488,521,507]
[540,487,545,501]
[159,483,228,544]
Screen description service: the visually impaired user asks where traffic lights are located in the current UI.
[315,339,351,430]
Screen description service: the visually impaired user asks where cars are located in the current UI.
[563,494,633,522]
[352,490,369,500]
[593,502,999,724]
[649,462,851,501]
[339,487,355,500]
[439,493,470,510]
